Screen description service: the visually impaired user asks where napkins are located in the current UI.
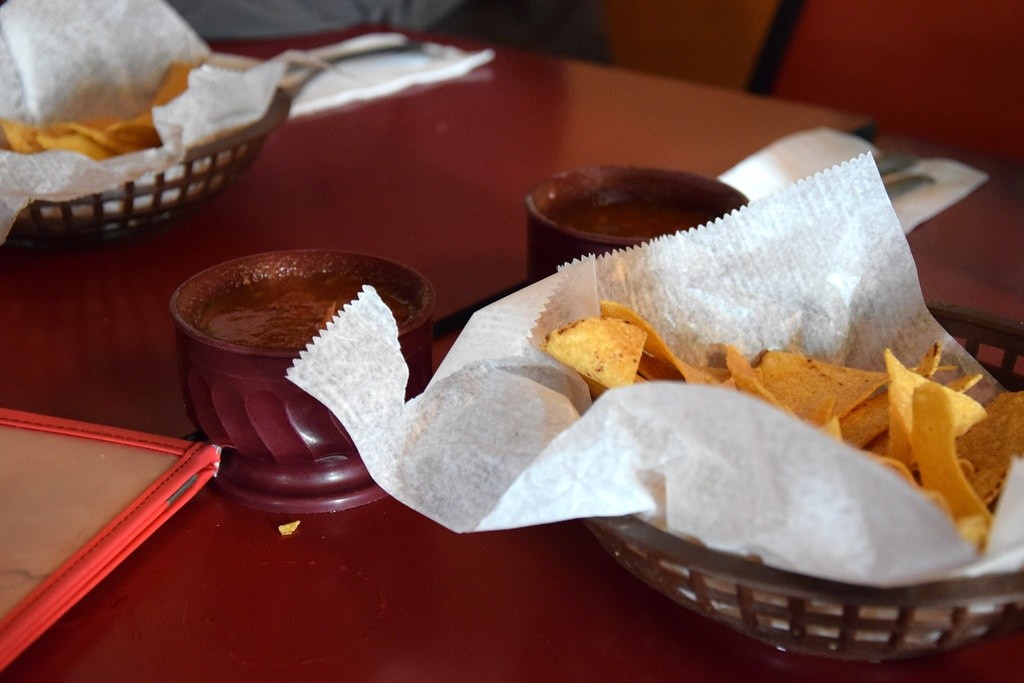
[288,32,495,119]
[716,124,990,234]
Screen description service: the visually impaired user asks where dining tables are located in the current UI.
[1,24,1023,683]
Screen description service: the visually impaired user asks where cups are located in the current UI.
[525,165,749,282]
[171,248,434,514]
